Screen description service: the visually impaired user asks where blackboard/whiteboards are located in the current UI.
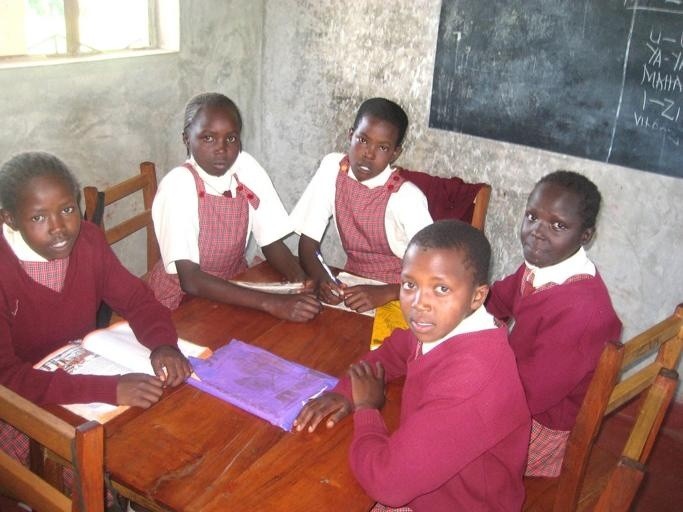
[429,0,682,179]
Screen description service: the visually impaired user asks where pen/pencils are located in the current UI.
[314,249,340,288]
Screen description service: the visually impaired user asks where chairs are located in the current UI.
[394,168,491,231]
[1,382,106,511]
[594,366,680,511]
[82,160,165,330]
[529,302,683,512]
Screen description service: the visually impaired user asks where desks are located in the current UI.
[11,253,521,511]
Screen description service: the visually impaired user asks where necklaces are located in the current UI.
[203,175,233,199]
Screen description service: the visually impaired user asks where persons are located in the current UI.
[0,149,195,511]
[149,90,327,326]
[485,169,623,479]
[292,218,534,511]
[289,96,435,315]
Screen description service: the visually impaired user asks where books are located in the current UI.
[369,299,415,351]
[225,279,310,295]
[317,265,389,317]
[31,317,214,423]
[184,334,337,434]
